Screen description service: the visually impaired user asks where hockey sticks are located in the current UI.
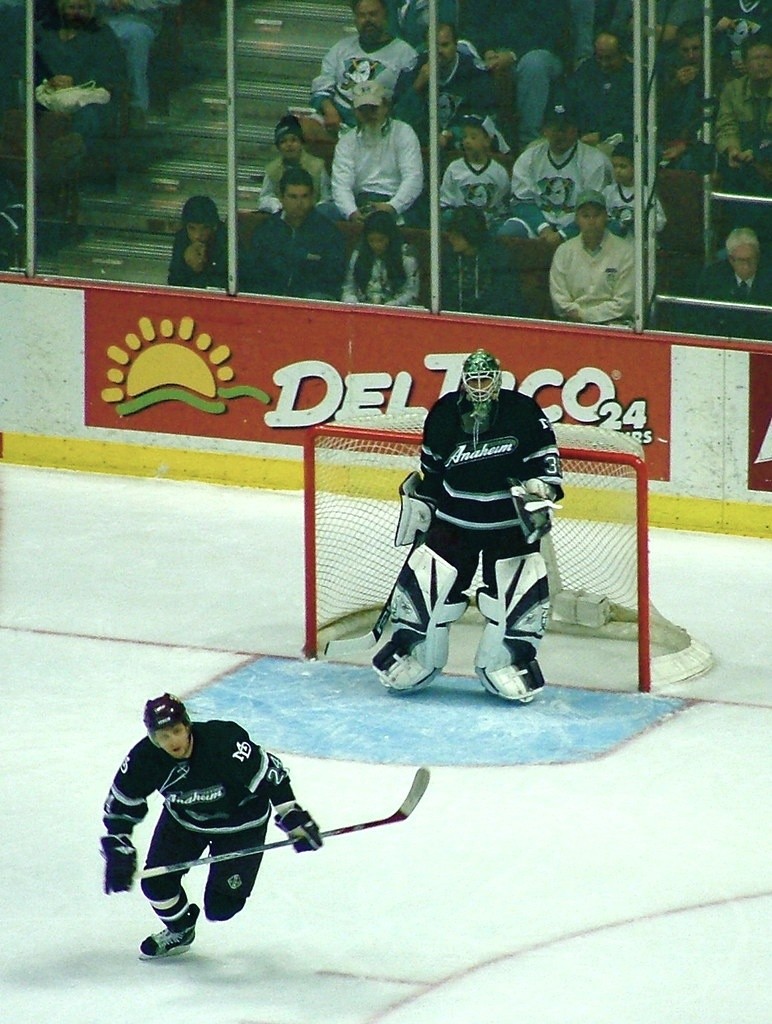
[131,766,431,879]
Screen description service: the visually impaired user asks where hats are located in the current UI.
[575,190,606,210]
[352,83,383,108]
[544,92,578,124]
[273,115,306,144]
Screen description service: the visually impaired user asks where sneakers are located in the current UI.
[138,903,200,962]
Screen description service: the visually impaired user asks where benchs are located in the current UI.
[227,139,723,323]
[1,1,184,230]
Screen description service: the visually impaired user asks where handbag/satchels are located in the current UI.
[37,77,110,113]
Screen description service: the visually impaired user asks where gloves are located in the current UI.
[99,833,138,896]
[274,802,323,854]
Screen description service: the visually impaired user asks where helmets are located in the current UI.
[143,693,192,739]
[461,348,503,405]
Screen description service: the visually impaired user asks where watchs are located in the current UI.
[442,130,447,135]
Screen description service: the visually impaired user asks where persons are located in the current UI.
[0,0,220,269]
[371,346,566,703]
[171,0,772,339]
[100,692,324,961]
